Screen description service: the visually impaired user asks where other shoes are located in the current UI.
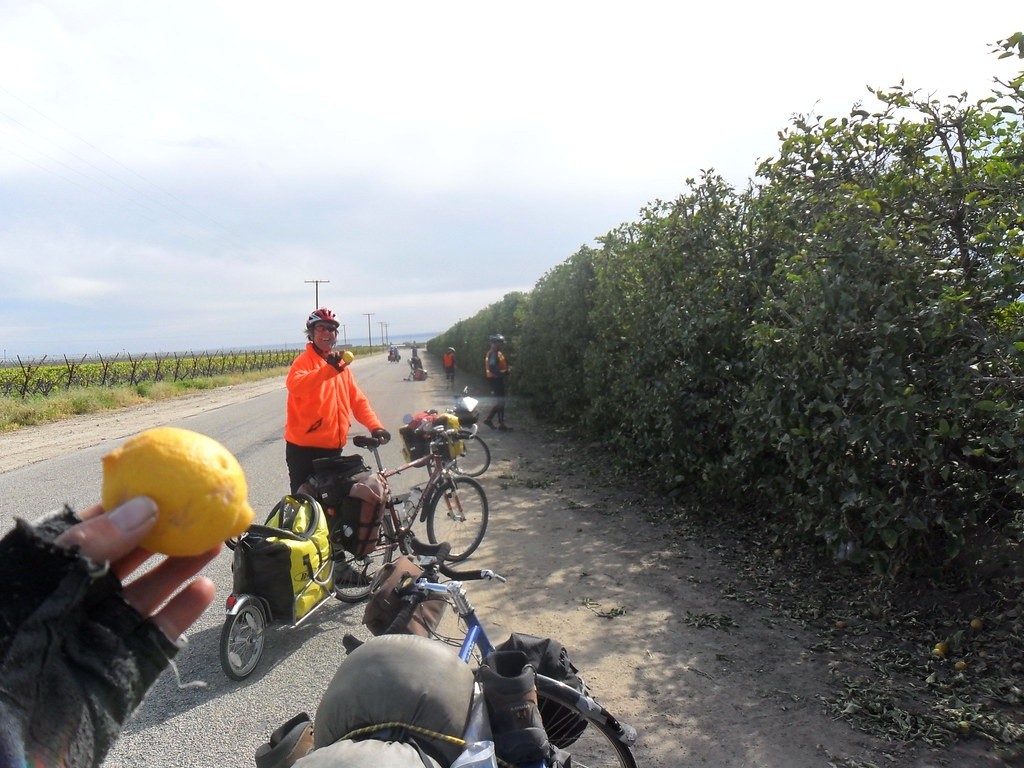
[336,564,374,584]
[485,420,496,430]
[498,423,514,431]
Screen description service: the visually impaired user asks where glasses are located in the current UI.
[311,324,337,332]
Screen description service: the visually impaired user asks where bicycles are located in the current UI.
[296,426,489,603]
[342,538,638,768]
[400,384,491,478]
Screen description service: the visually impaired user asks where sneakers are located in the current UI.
[256,712,318,768]
[479,650,550,765]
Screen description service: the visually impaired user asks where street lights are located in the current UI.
[376,321,392,353]
[305,280,330,310]
[362,314,374,355]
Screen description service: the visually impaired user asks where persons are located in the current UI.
[390,347,395,362]
[443,346,456,391]
[393,346,400,362]
[0,496,222,767]
[411,341,420,357]
[485,335,513,431]
[285,307,391,584]
[408,366,423,381]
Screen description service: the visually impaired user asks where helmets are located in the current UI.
[449,347,456,352]
[306,309,339,328]
[489,333,505,343]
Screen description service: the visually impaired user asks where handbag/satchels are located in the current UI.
[297,456,390,560]
[234,494,334,621]
[399,414,464,466]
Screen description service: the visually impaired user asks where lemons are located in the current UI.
[99,426,254,557]
[342,351,354,364]
